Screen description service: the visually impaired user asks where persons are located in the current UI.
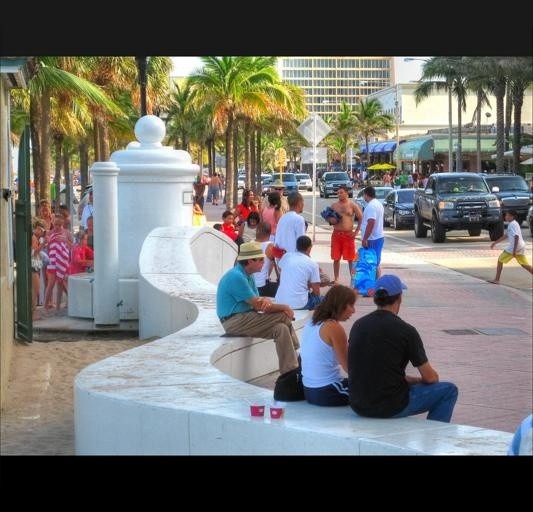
[192,171,224,216]
[214,240,300,377]
[487,209,533,286]
[360,187,384,277]
[301,282,357,410]
[345,273,460,426]
[215,189,306,296]
[328,185,363,282]
[31,187,94,321]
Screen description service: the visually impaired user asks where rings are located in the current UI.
[268,303,270,305]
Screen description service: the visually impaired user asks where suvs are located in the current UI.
[318,171,353,199]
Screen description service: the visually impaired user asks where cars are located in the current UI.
[234,172,312,196]
[351,172,532,245]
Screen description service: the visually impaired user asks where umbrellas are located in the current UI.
[366,163,396,176]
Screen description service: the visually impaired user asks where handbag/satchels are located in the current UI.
[274,367,305,402]
[350,246,378,297]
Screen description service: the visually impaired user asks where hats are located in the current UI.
[268,179,287,188]
[237,241,266,261]
[373,274,409,298]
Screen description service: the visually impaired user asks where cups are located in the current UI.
[250,403,263,416]
[256,301,264,314]
[270,407,285,418]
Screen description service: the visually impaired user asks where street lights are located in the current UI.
[321,98,353,174]
[403,57,454,173]
[357,80,399,174]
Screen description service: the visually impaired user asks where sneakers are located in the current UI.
[486,278,501,285]
